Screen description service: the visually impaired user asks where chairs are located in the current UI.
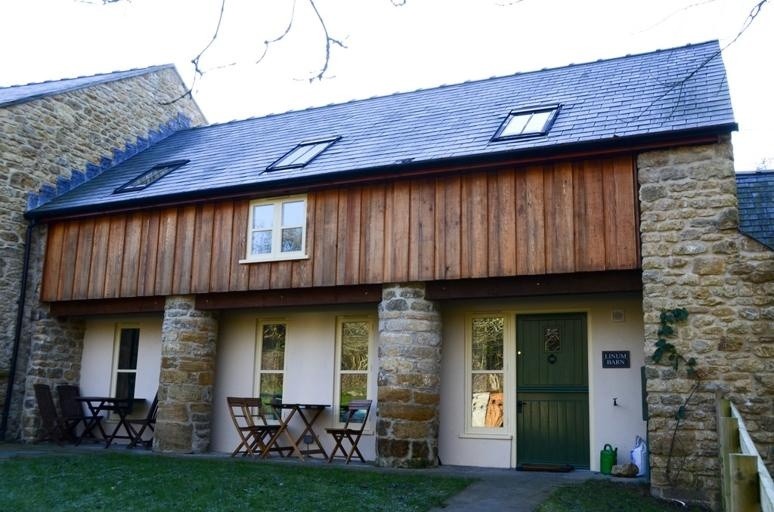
[33,384,158,449]
[226,396,372,464]
